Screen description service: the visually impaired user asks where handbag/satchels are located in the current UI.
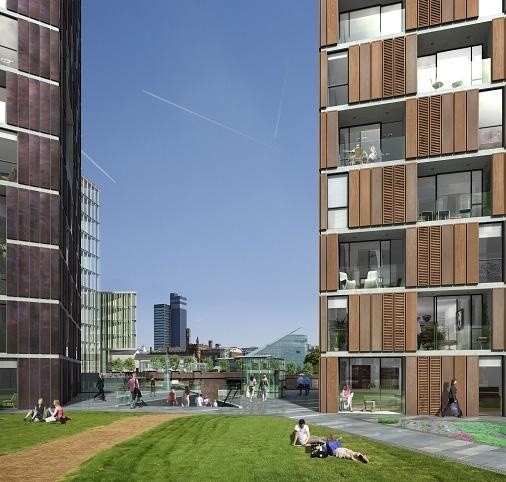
[450,403,458,415]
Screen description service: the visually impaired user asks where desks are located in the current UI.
[342,149,368,165]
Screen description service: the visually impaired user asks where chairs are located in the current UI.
[339,271,356,290]
[389,277,402,288]
[363,271,379,289]
[349,149,363,166]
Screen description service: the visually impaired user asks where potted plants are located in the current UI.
[330,317,346,352]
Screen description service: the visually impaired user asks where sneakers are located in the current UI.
[357,455,368,463]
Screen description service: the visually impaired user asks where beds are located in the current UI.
[421,210,433,221]
